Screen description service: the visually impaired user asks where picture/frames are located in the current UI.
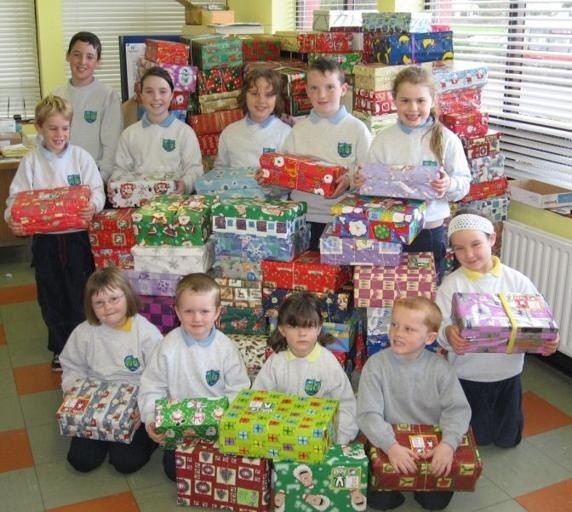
[118,34,179,102]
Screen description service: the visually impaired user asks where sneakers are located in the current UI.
[50,350,62,373]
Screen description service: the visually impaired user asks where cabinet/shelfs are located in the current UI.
[0,141,30,247]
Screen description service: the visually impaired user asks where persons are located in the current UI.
[251,293,360,446]
[254,57,371,250]
[3,96,106,370]
[214,62,291,195]
[356,297,472,512]
[108,67,204,205]
[40,31,125,181]
[435,208,560,449]
[136,273,249,481]
[57,266,163,476]
[363,67,470,282]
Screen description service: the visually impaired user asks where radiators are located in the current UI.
[500,219,572,356]
